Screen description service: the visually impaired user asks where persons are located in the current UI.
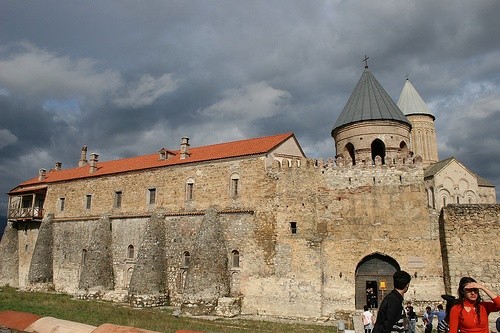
[432,304,445,321]
[368,288,377,310]
[361,305,375,333]
[438,277,500,333]
[372,271,411,333]
[406,302,416,333]
[423,307,433,333]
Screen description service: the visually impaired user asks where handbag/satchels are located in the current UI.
[438,300,462,333]
